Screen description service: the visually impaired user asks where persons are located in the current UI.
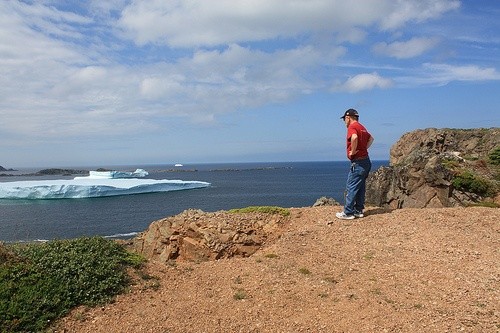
[335,107,374,220]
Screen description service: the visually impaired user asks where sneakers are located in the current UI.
[335,211,355,219]
[352,209,364,218]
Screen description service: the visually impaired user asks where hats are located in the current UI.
[340,108,359,118]
